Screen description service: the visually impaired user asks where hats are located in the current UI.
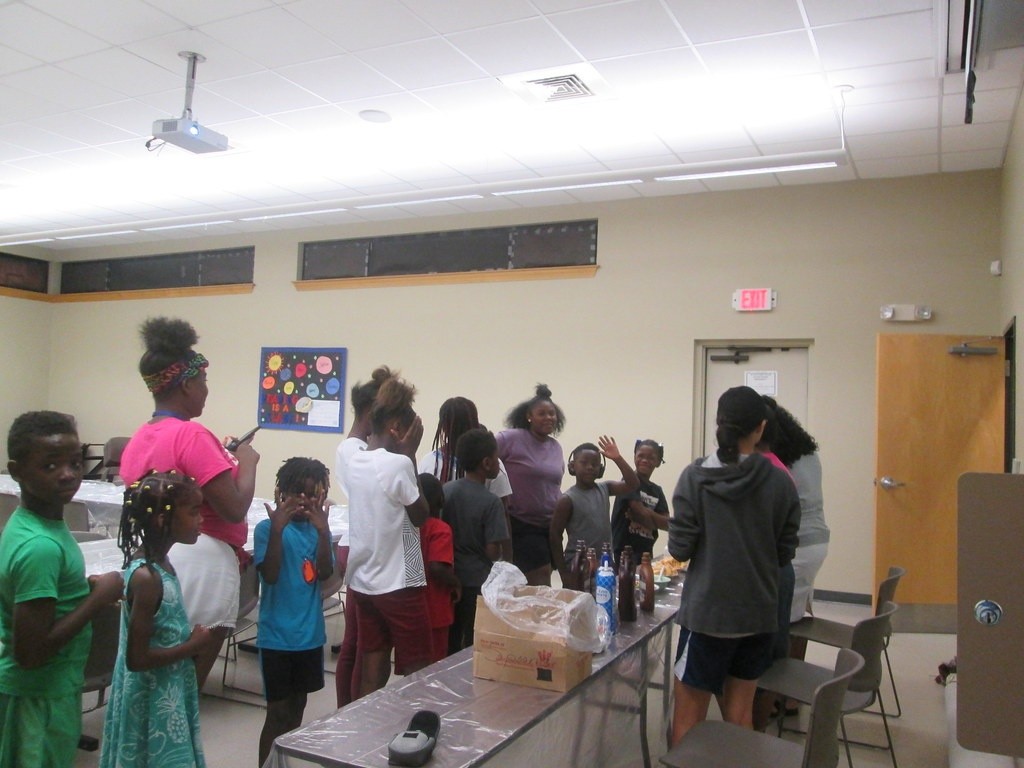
[717,386,769,427]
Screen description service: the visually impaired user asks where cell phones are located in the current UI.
[232,425,262,452]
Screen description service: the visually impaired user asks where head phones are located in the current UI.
[568,450,606,478]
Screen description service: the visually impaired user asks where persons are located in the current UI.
[333,363,832,753]
[118,316,260,714]
[252,455,334,768]
[98,466,211,768]
[0,409,125,768]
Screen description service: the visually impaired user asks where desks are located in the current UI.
[0,476,689,768]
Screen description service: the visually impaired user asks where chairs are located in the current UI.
[755,600,899,768]
[216,561,258,687]
[319,545,349,651]
[80,601,119,718]
[658,648,865,768]
[789,566,906,751]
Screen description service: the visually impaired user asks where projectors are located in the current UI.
[153,119,228,154]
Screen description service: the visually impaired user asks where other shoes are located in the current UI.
[768,700,799,718]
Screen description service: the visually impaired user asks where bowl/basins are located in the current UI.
[676,570,687,583]
[616,574,671,596]
[666,575,681,584]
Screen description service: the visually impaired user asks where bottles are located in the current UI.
[569,541,598,592]
[619,546,637,622]
[601,543,615,564]
[596,561,616,637]
[639,553,654,612]
[595,554,615,624]
